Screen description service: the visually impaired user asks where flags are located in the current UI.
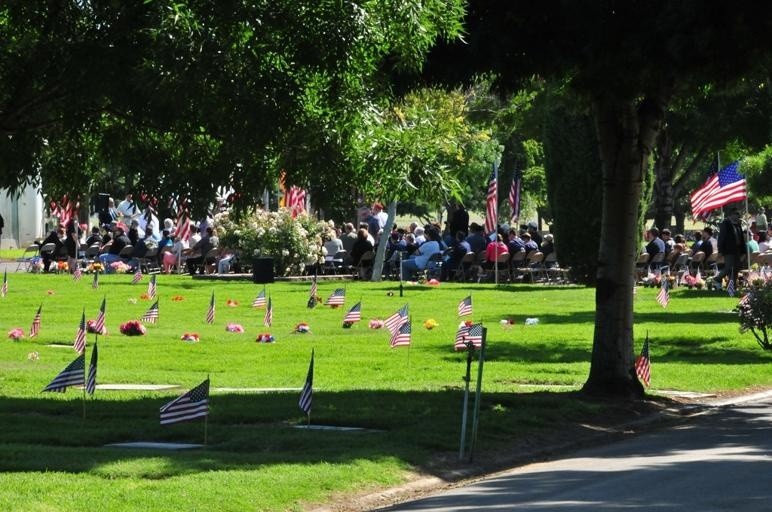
[74,300,106,353]
[298,348,315,416]
[158,377,212,426]
[250,288,272,328]
[481,159,499,241]
[636,331,652,389]
[207,294,215,324]
[455,296,482,352]
[508,155,523,226]
[320,202,556,285]
[656,276,669,309]
[737,289,760,328]
[382,304,411,348]
[39,346,88,396]
[310,275,360,323]
[689,157,748,223]
[29,302,43,339]
[172,192,190,241]
[73,263,99,288]
[129,268,163,325]
[2,268,7,296]
[86,341,99,396]
[48,193,73,226]
[282,184,305,217]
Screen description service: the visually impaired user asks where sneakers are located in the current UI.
[712,279,721,290]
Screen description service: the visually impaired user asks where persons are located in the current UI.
[636,206,772,289]
[33,215,244,274]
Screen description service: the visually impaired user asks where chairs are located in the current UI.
[13,240,772,286]
